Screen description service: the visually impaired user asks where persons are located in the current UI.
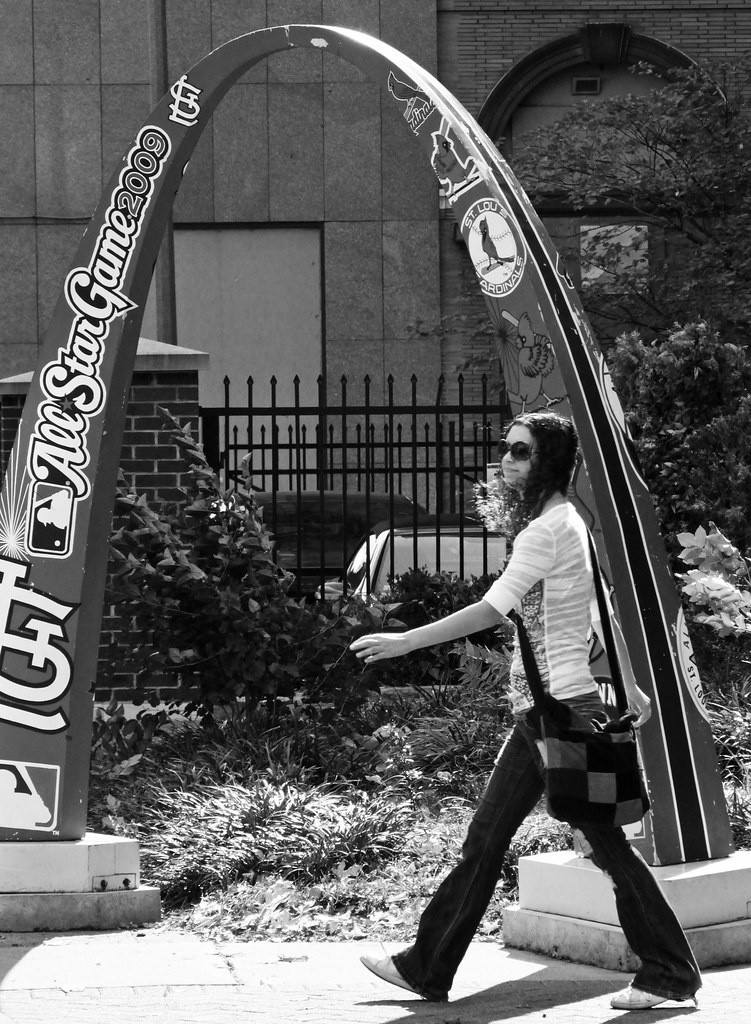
[348,416,706,1011]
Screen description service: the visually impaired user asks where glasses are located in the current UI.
[496,438,542,463]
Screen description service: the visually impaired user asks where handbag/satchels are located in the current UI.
[533,693,650,828]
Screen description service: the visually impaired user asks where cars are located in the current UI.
[317,521,516,623]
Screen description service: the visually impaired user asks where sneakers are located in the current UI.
[358,951,427,1002]
[610,982,671,1010]
